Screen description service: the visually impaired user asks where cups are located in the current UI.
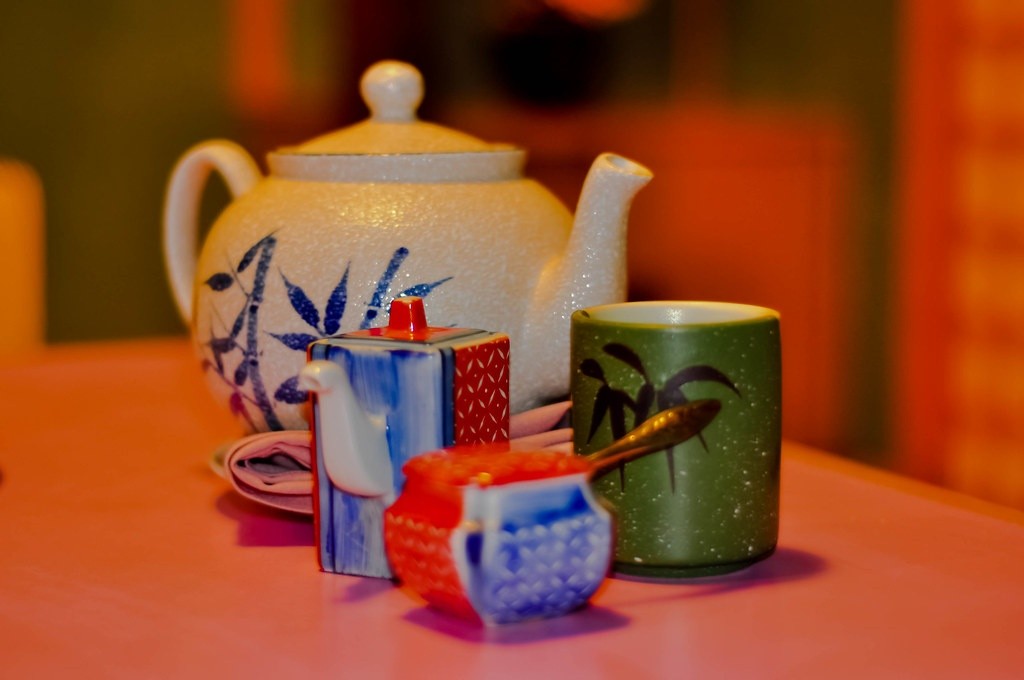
[569,300,781,578]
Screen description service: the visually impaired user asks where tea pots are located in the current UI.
[162,61,655,436]
